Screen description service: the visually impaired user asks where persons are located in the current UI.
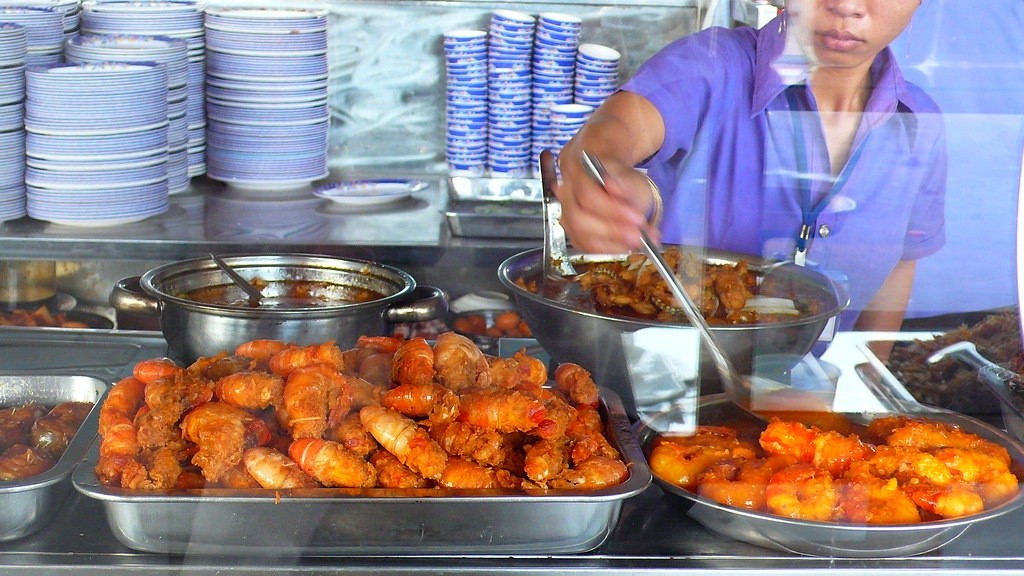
[558,0,947,412]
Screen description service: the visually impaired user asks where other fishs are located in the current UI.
[511,244,823,327]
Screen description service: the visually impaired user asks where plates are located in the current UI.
[0,2,329,230]
[634,391,1024,558]
[312,177,427,204]
[449,290,520,313]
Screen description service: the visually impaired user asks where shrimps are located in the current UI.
[647,411,1022,526]
[0,398,95,482]
[93,330,631,493]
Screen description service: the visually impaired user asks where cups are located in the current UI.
[443,9,620,179]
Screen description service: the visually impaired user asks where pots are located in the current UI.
[109,253,447,365]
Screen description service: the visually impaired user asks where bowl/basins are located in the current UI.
[498,241,851,406]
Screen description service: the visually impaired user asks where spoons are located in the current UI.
[926,341,1022,396]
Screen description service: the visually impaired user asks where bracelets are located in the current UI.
[643,174,663,232]
